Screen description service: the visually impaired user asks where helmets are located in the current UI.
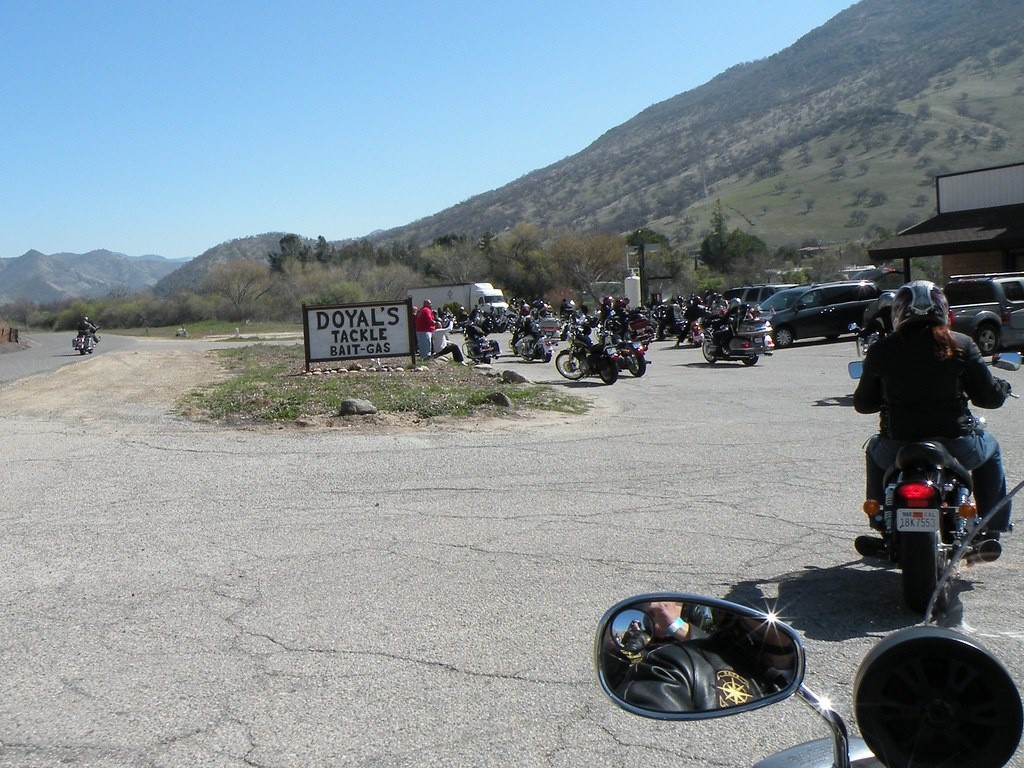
[456,305,465,314]
[603,296,614,307]
[891,280,948,333]
[675,296,684,304]
[604,319,622,335]
[82,316,88,322]
[693,297,702,303]
[532,301,539,308]
[564,299,575,311]
[615,297,626,308]
[711,607,796,671]
[877,292,896,310]
[510,298,517,305]
[729,298,741,310]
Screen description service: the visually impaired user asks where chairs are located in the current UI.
[786,297,805,311]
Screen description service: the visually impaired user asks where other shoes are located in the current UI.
[98,336,101,343]
[457,361,469,366]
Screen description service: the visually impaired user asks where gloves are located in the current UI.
[998,378,1011,395]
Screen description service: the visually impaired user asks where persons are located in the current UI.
[602,602,797,713]
[854,280,1011,536]
[433,318,468,366]
[415,300,436,360]
[77,317,101,344]
[413,290,744,355]
[854,292,895,342]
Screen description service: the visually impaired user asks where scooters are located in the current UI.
[591,589,1024,768]
[849,322,885,358]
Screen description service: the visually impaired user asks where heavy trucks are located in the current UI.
[406,282,510,316]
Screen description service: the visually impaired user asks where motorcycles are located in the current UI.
[846,353,1024,611]
[72,326,101,355]
[438,301,730,378]
[702,311,774,367]
[555,317,622,386]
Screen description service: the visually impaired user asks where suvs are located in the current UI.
[758,279,885,347]
[930,272,1024,356]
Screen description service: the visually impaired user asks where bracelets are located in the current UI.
[667,618,683,635]
